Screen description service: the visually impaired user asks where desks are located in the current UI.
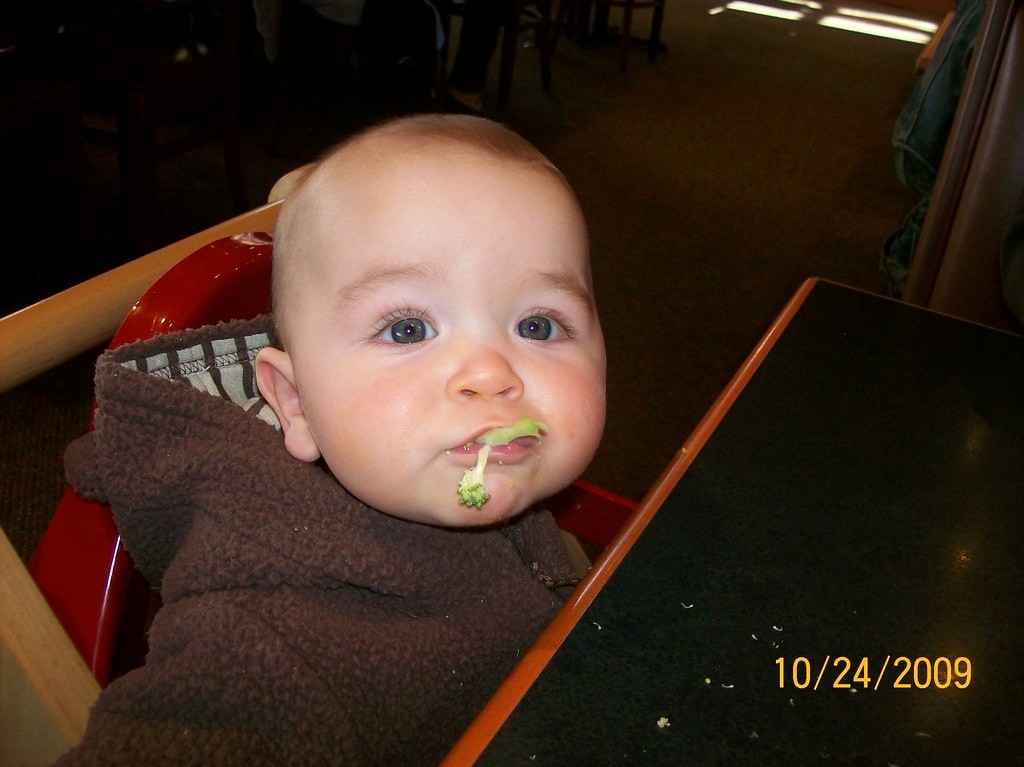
[425,276,1024,767]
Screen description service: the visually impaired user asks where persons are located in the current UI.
[48,114,607,767]
[254,0,445,95]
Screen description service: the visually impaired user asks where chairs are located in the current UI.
[0,158,644,767]
